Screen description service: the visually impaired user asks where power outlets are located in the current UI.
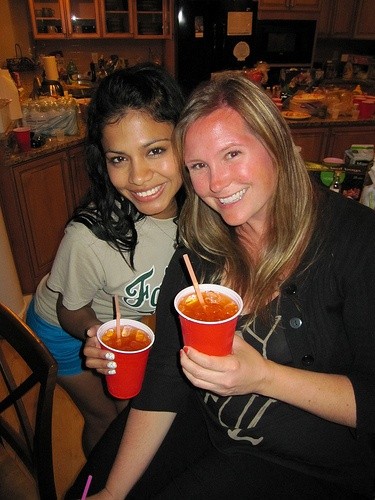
[92,53,102,63]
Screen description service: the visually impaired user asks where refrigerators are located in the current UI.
[174,0,259,98]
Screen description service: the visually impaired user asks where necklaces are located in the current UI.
[148,216,179,250]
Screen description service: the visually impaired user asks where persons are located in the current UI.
[26,61,192,462]
[64,75,375,500]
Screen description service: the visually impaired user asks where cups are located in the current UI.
[173,283,244,357]
[12,127,31,151]
[32,7,62,34]
[97,319,155,399]
[56,129,65,141]
[322,86,375,121]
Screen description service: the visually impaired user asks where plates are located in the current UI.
[279,92,324,121]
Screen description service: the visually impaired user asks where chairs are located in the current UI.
[0,302,58,500]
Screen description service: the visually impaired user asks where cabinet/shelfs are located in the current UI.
[256,0,375,40]
[0,143,91,295]
[28,0,173,40]
[289,125,375,163]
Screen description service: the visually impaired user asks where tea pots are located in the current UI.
[33,77,65,96]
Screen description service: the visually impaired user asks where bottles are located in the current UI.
[67,58,78,84]
[18,94,79,138]
